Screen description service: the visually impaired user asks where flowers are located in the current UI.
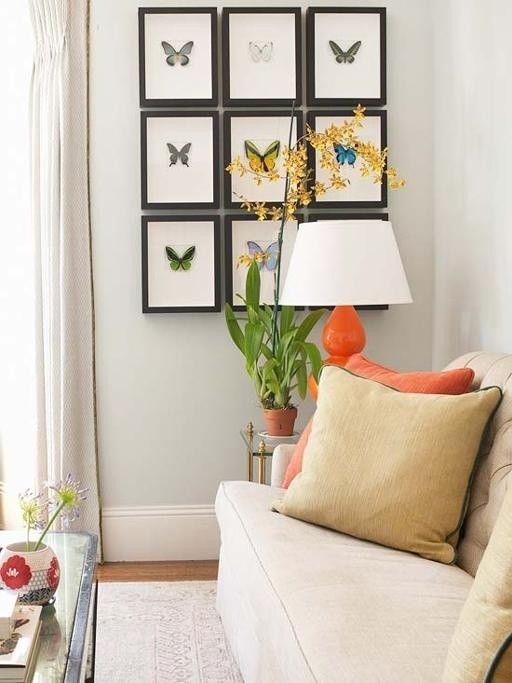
[224,102,409,408]
[18,474,87,552]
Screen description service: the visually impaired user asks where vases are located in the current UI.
[260,409,297,438]
[0,540,60,605]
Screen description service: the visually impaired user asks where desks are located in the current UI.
[242,422,302,484]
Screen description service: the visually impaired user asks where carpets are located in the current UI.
[93,579,240,683]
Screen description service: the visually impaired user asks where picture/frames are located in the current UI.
[140,111,220,210]
[224,212,302,311]
[222,6,302,107]
[307,6,386,107]
[309,213,390,310]
[307,109,387,209]
[138,6,219,109]
[224,110,302,209]
[142,215,222,313]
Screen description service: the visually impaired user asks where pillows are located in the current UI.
[281,355,474,489]
[276,363,503,567]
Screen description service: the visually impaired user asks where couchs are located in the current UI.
[213,347,511,683]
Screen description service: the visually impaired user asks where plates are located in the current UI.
[258,429,299,439]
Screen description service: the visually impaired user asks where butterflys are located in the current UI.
[166,246,195,271]
[247,241,279,271]
[161,40,194,67]
[245,139,280,172]
[249,40,274,63]
[166,142,192,167]
[335,143,356,169]
[329,40,362,64]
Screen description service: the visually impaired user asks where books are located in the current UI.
[0,589,43,683]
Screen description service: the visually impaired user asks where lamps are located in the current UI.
[275,218,413,402]
